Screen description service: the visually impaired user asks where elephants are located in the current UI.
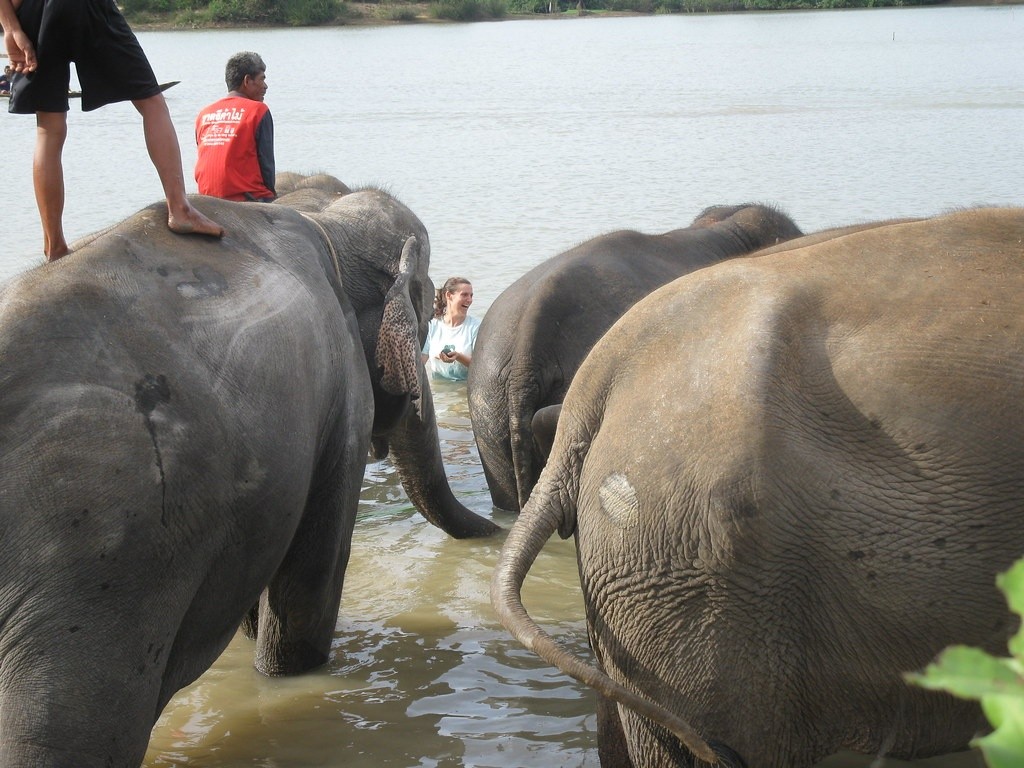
[466,199,1022,768]
[1,165,511,768]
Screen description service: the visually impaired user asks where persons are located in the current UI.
[0,0,227,264]
[195,51,276,202]
[424,277,482,382]
[0,66,10,94]
